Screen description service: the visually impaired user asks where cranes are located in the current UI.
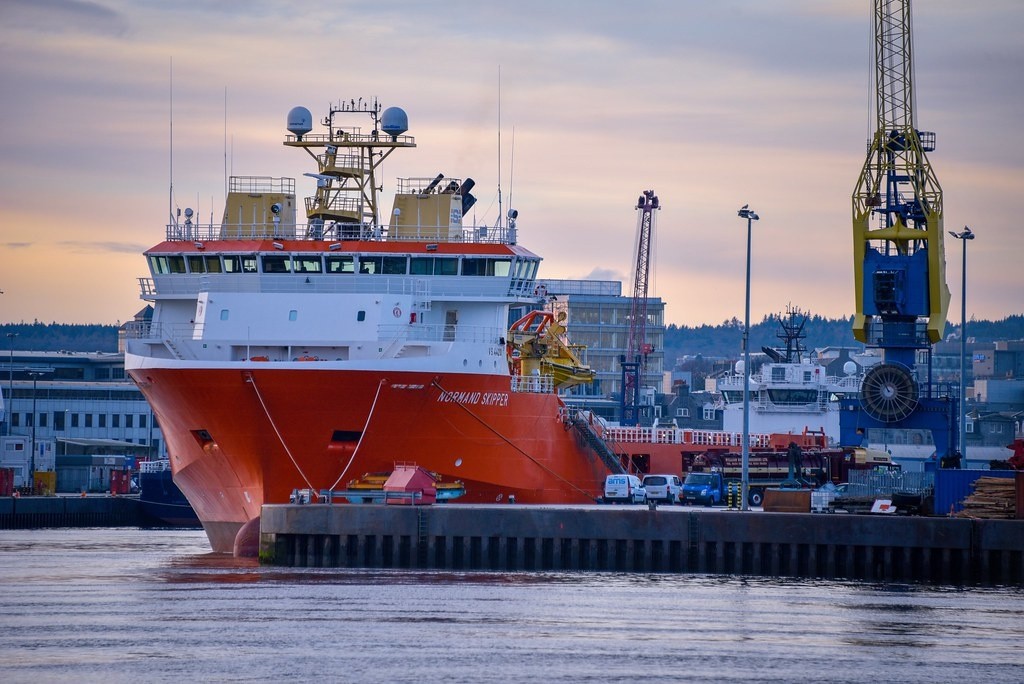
[624,190,661,419]
[838,0,960,466]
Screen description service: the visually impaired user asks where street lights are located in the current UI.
[738,209,759,511]
[7,332,21,436]
[27,372,46,495]
[947,230,975,470]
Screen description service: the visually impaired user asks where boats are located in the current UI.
[716,301,881,446]
[123,58,829,561]
[134,457,203,528]
[505,310,597,388]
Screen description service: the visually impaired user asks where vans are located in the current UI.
[641,475,685,505]
[833,483,868,500]
[604,474,648,505]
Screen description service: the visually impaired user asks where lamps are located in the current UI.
[329,243,341,250]
[184,208,193,219]
[426,244,438,250]
[194,242,204,248]
[507,209,518,222]
[273,243,283,249]
[271,202,282,217]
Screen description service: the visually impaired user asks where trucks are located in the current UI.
[678,471,816,508]
